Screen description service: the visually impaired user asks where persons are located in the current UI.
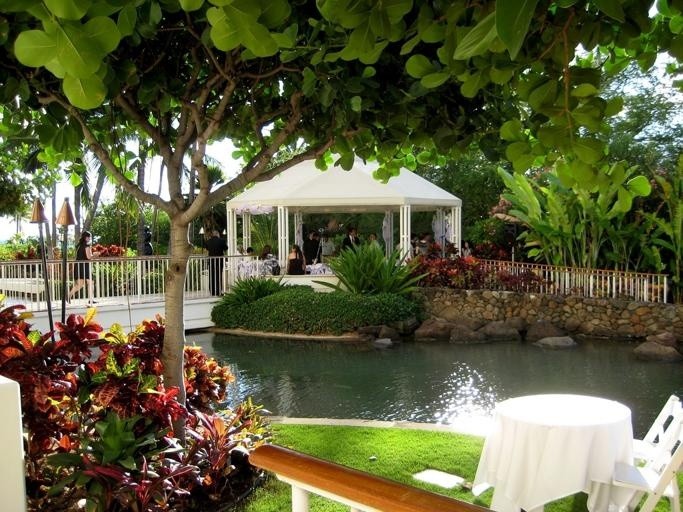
[461,239,473,257]
[301,227,361,265]
[205,230,229,297]
[143,225,154,256]
[395,232,434,262]
[256,245,278,276]
[243,246,257,274]
[285,244,306,275]
[65,229,101,305]
[367,232,378,243]
[236,244,244,272]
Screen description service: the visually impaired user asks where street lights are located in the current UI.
[27,194,56,342]
[51,195,79,323]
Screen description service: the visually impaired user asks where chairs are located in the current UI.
[612,394,683,512]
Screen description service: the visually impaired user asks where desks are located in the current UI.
[472,393,634,511]
